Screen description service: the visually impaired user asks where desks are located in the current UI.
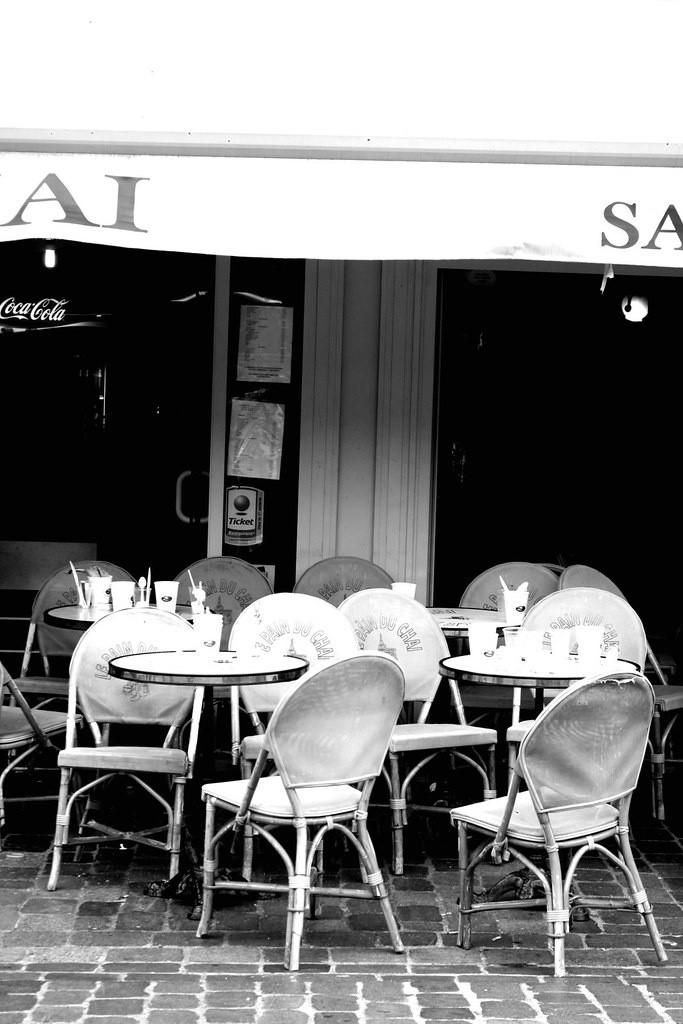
[42,601,216,631]
[437,649,641,922]
[107,650,309,922]
[425,606,521,656]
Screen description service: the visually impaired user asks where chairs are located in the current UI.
[1,558,683,972]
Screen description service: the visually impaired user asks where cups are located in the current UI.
[467,633,500,663]
[154,581,180,614]
[391,582,416,601]
[549,629,571,658]
[192,614,223,658]
[502,628,523,656]
[87,575,113,607]
[502,591,529,623]
[606,644,618,666]
[74,580,93,609]
[497,589,506,617]
[132,587,152,607]
[110,581,135,611]
[573,625,602,669]
[518,631,541,662]
[187,586,207,614]
